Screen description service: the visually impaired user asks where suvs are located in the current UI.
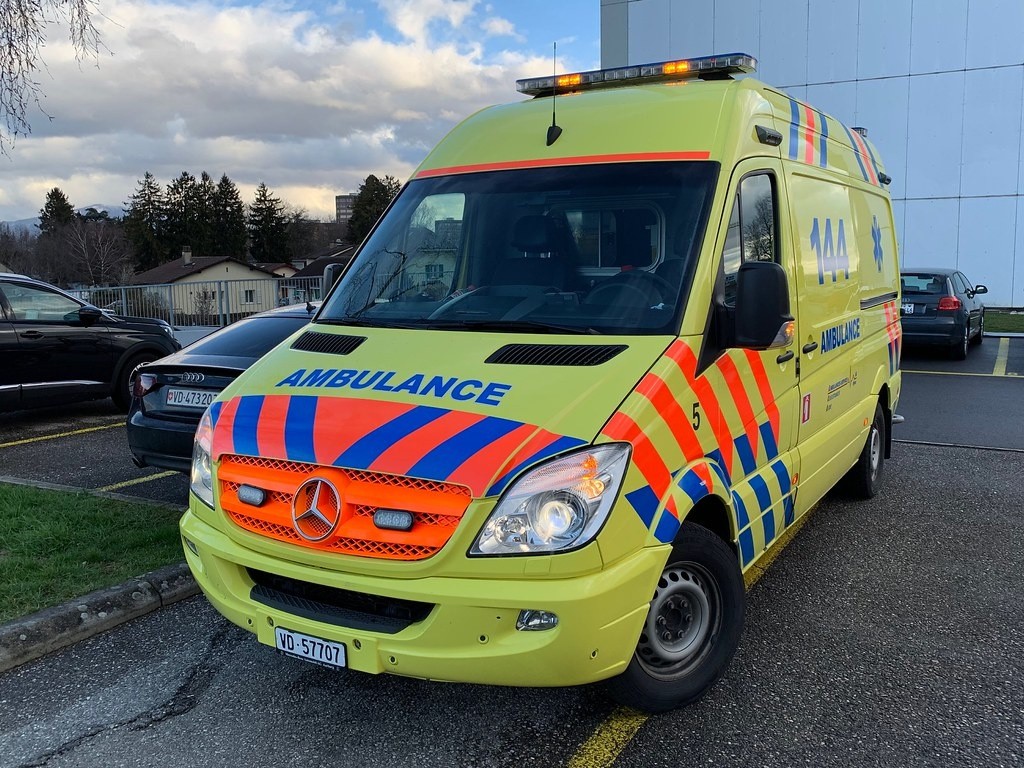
[0,272,184,414]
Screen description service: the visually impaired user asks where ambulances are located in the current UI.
[176,51,902,721]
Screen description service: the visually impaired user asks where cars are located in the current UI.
[899,267,989,361]
[123,302,335,475]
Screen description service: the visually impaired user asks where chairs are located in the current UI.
[901,278,905,291]
[929,278,941,292]
[650,218,697,303]
[489,215,579,293]
